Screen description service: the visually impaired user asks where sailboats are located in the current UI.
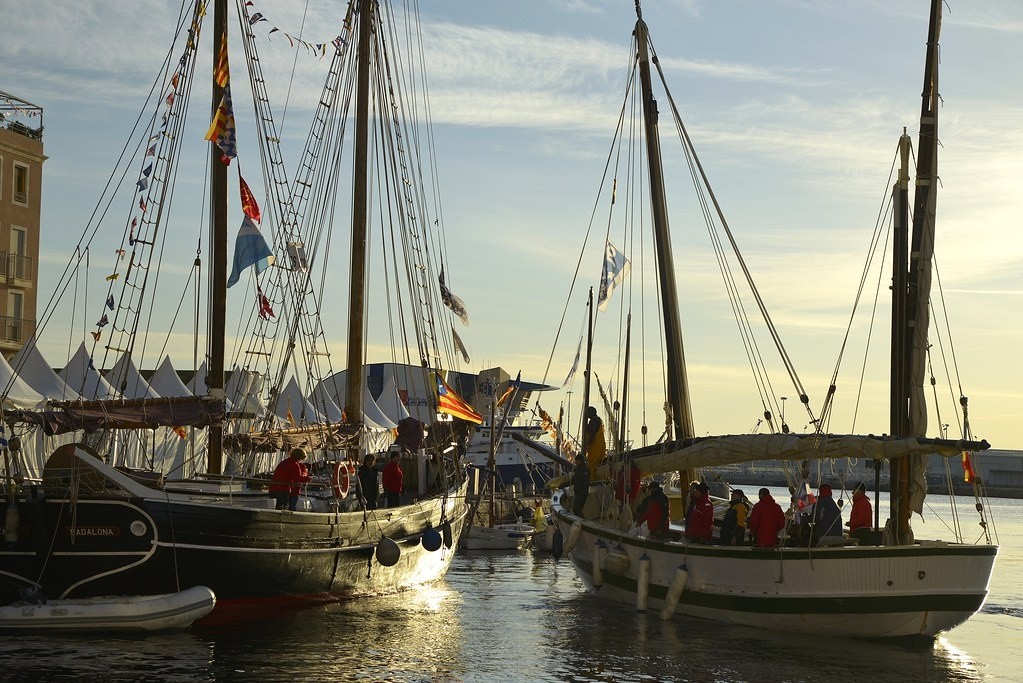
[0,2,1001,636]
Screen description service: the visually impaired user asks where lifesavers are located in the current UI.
[334,462,350,499]
[617,466,641,502]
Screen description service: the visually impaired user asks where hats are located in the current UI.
[646,481,659,488]
[730,489,744,496]
[694,482,710,494]
[855,482,867,491]
[820,485,832,496]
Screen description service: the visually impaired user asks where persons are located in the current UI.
[812,484,842,536]
[750,488,784,546]
[635,481,669,539]
[719,489,749,546]
[381,451,403,506]
[356,453,378,510]
[684,481,715,544]
[585,406,605,481]
[269,448,312,511]
[846,482,872,536]
[558,454,590,519]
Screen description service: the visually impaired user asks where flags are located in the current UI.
[439,269,469,326]
[204,28,274,288]
[91,0,207,341]
[963,450,975,484]
[452,328,470,364]
[598,240,630,313]
[168,425,187,440]
[497,382,513,408]
[287,411,294,426]
[435,371,482,424]
